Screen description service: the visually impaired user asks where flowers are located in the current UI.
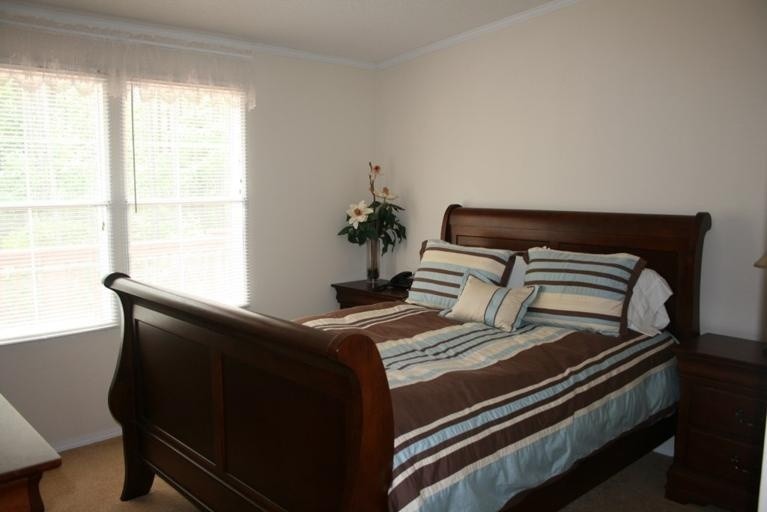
[338,161,405,257]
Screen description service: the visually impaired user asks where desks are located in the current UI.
[1,394,62,512]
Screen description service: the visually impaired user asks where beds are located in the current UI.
[105,205,711,512]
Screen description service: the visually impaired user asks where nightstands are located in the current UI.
[665,333,767,512]
[331,280,408,309]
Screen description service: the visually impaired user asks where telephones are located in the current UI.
[391,271,414,290]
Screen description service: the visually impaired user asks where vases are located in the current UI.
[366,240,384,284]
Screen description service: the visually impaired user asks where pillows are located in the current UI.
[404,239,674,338]
